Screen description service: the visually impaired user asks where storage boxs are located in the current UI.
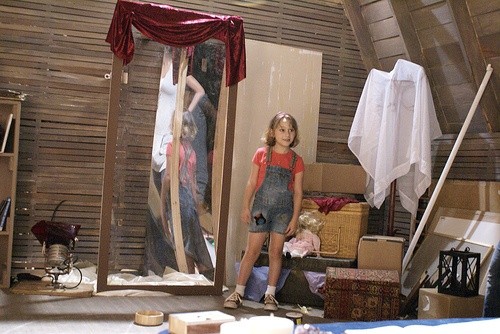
[288,197,370,259]
[241,248,356,308]
[168,310,235,334]
[323,267,401,321]
[302,162,368,193]
[358,235,405,275]
[418,288,486,318]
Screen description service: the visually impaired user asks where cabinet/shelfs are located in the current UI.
[0,96,22,288]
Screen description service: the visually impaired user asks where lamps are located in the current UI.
[32,221,81,288]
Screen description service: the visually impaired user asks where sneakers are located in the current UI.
[224,292,243,308]
[264,294,279,311]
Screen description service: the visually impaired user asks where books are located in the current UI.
[0,114,14,154]
[0,197,11,232]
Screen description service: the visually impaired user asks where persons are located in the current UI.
[222,112,306,312]
[151,47,214,282]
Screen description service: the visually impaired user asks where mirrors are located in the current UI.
[99,2,240,291]
